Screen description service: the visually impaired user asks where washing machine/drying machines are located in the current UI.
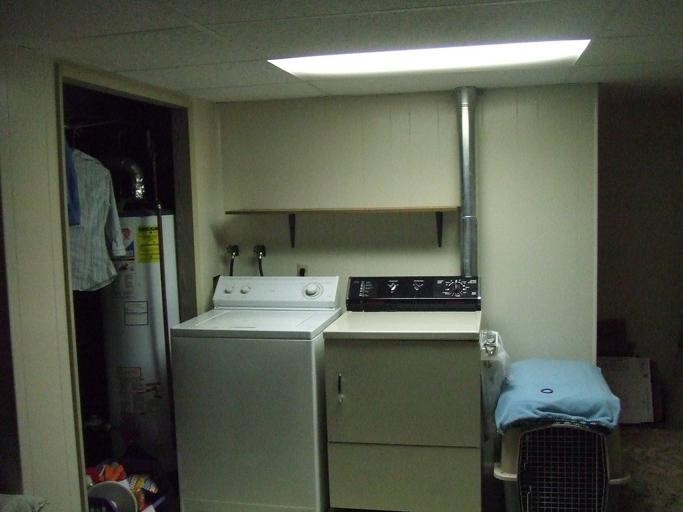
[167,274,342,512]
[322,274,485,512]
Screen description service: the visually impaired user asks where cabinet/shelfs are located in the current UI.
[324,336,481,511]
